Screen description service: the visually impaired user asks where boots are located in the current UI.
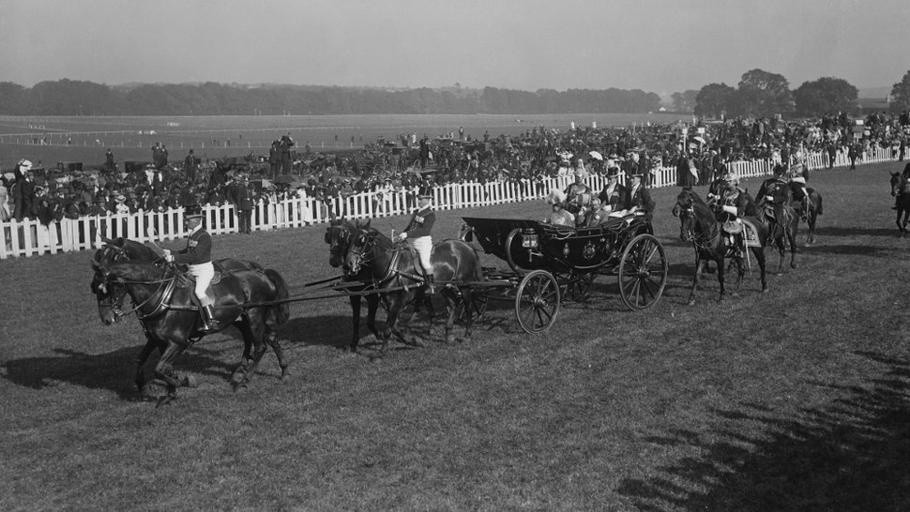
[198,305,220,333]
[424,274,435,296]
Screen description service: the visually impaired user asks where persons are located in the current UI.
[161,205,219,335]
[398,183,442,295]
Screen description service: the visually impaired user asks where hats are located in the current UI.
[185,206,205,220]
[606,166,619,179]
[774,166,783,176]
[724,173,739,186]
[720,165,726,175]
[630,165,644,177]
[416,186,431,199]
[573,168,582,178]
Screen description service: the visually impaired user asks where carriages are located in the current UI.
[90,213,669,406]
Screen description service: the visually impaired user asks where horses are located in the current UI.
[888,169,910,241]
[327,210,486,365]
[669,175,823,308]
[90,230,292,410]
